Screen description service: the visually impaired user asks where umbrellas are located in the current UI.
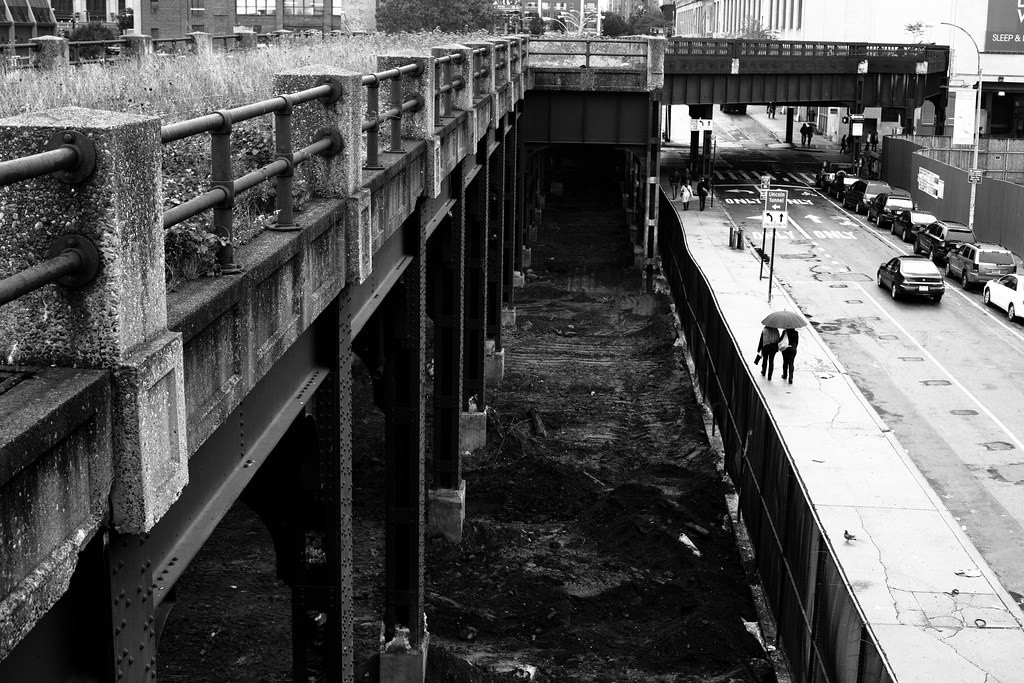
[761,309,807,329]
[808,122,817,127]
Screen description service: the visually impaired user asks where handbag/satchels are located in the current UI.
[777,327,789,351]
[754,354,761,365]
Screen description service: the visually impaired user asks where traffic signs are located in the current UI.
[764,190,788,229]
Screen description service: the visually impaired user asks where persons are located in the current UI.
[838,130,879,155]
[780,328,799,384]
[680,169,692,187]
[758,325,780,381]
[766,105,775,119]
[669,169,681,200]
[697,178,710,210]
[680,180,694,211]
[800,124,813,148]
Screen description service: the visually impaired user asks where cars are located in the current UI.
[827,175,863,201]
[720,104,747,115]
[983,273,1024,322]
[877,254,945,302]
[891,210,937,243]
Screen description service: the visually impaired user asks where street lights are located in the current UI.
[926,22,982,230]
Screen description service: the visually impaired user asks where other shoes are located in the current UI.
[761,370,765,376]
[782,375,786,379]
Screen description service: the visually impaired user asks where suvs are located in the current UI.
[867,192,916,228]
[913,219,978,264]
[842,180,892,214]
[944,242,1018,291]
[815,160,853,192]
